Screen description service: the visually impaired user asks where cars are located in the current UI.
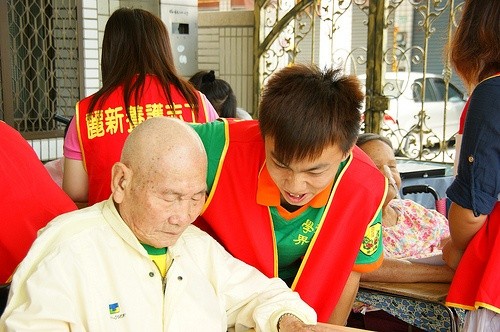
[352,71,469,156]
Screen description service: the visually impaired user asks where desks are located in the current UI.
[393,159,461,213]
[355,282,467,332]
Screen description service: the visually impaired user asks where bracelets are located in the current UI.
[277,313,304,332]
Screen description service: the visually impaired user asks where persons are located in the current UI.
[1,115,342,332]
[355,132,457,283]
[0,120,79,317]
[62,7,221,207]
[184,66,389,327]
[189,69,254,121]
[442,0,500,332]
